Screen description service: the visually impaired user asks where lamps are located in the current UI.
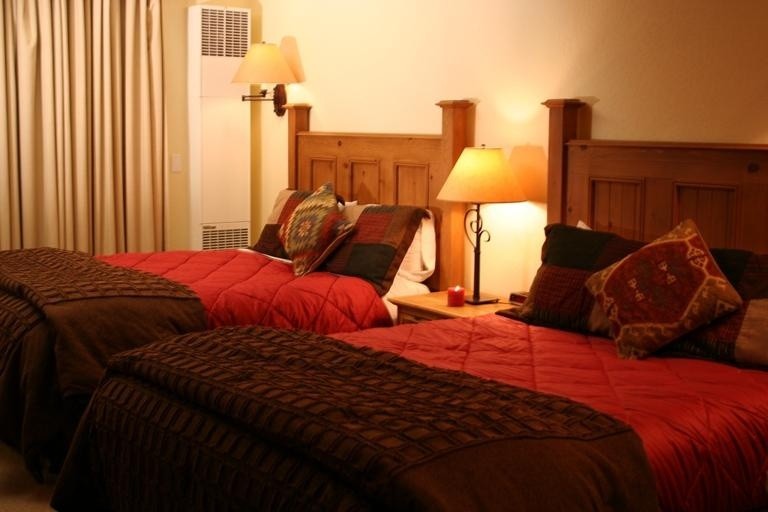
[230,39,295,120]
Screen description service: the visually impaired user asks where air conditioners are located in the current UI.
[184,4,253,256]
